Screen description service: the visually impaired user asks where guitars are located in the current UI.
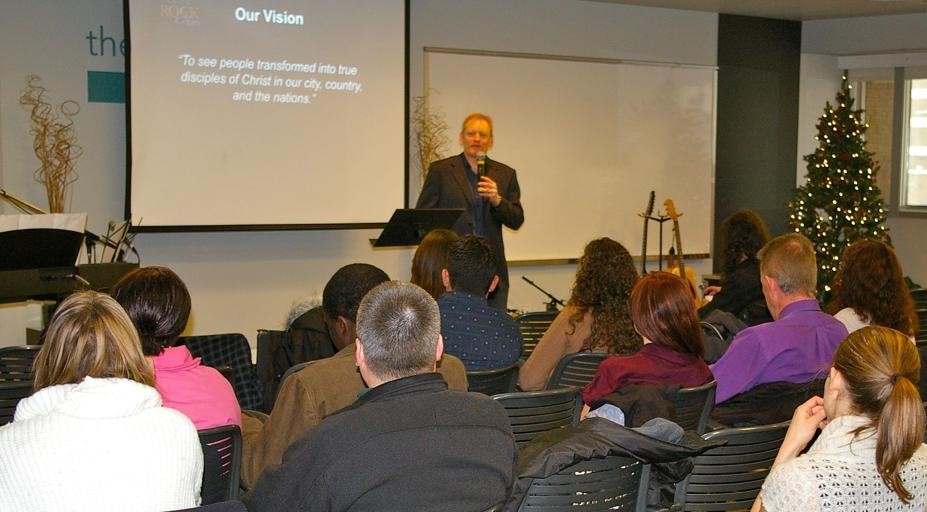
[663,198,704,310]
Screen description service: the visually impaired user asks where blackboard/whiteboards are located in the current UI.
[423,47,720,267]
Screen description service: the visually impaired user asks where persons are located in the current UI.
[823,239,920,347]
[748,325,927,512]
[1,290,206,512]
[516,237,644,390]
[708,232,850,406]
[696,209,775,343]
[237,262,469,490]
[409,228,459,301]
[435,235,523,392]
[246,280,518,512]
[577,270,716,424]
[415,113,524,314]
[110,264,245,478]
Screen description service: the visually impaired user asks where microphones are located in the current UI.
[476,151,487,206]
